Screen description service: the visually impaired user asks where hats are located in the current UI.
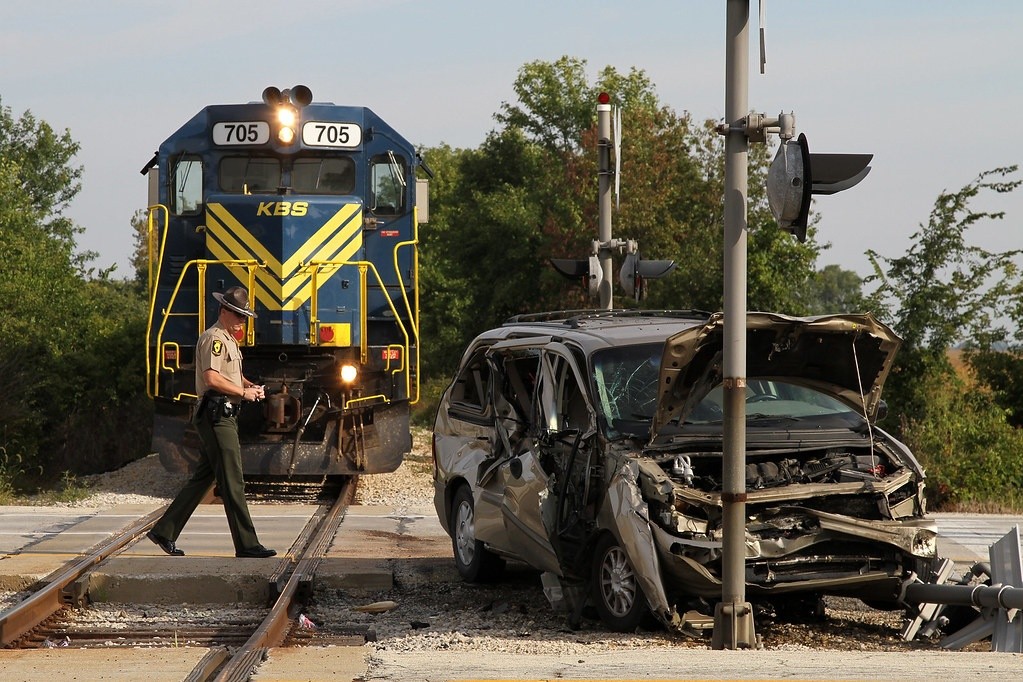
[212,287,258,318]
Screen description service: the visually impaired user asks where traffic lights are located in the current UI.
[620,251,678,303]
[766,133,876,243]
[548,256,604,296]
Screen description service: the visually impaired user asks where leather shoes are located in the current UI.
[235,544,276,558]
[146,532,184,556]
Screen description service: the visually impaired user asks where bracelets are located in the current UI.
[248,384,254,387]
[242,388,246,397]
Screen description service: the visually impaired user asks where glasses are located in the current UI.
[224,307,246,319]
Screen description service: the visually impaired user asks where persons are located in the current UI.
[146,287,277,558]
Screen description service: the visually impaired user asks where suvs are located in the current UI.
[432,307,945,637]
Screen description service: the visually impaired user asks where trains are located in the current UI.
[140,85,435,477]
